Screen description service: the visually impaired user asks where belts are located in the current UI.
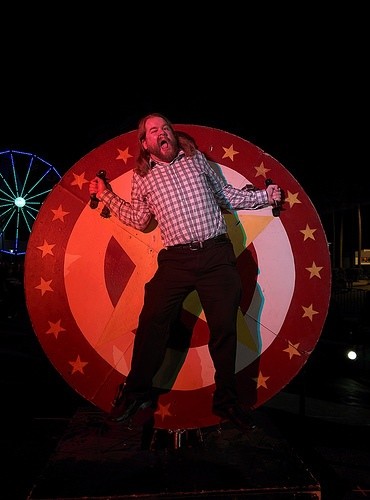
[168,233,229,251]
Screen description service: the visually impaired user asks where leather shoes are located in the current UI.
[213,406,258,433]
[111,399,149,422]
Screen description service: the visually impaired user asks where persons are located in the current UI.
[86,114,282,434]
